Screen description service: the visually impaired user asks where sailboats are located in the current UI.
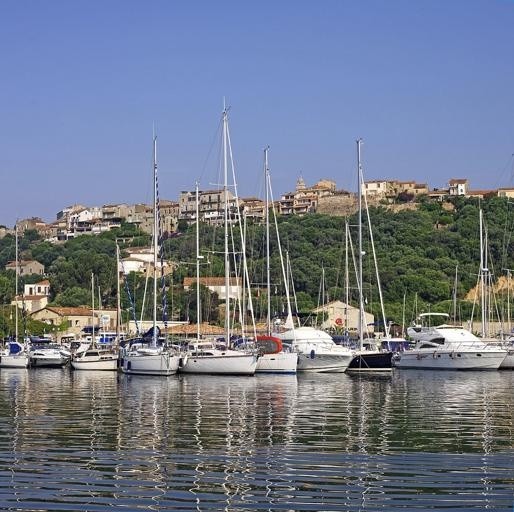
[254,145,298,374]
[120,119,181,376]
[340,136,395,377]
[181,100,259,376]
[0,96,514,379]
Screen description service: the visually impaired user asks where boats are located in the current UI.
[272,326,359,373]
[394,323,510,371]
